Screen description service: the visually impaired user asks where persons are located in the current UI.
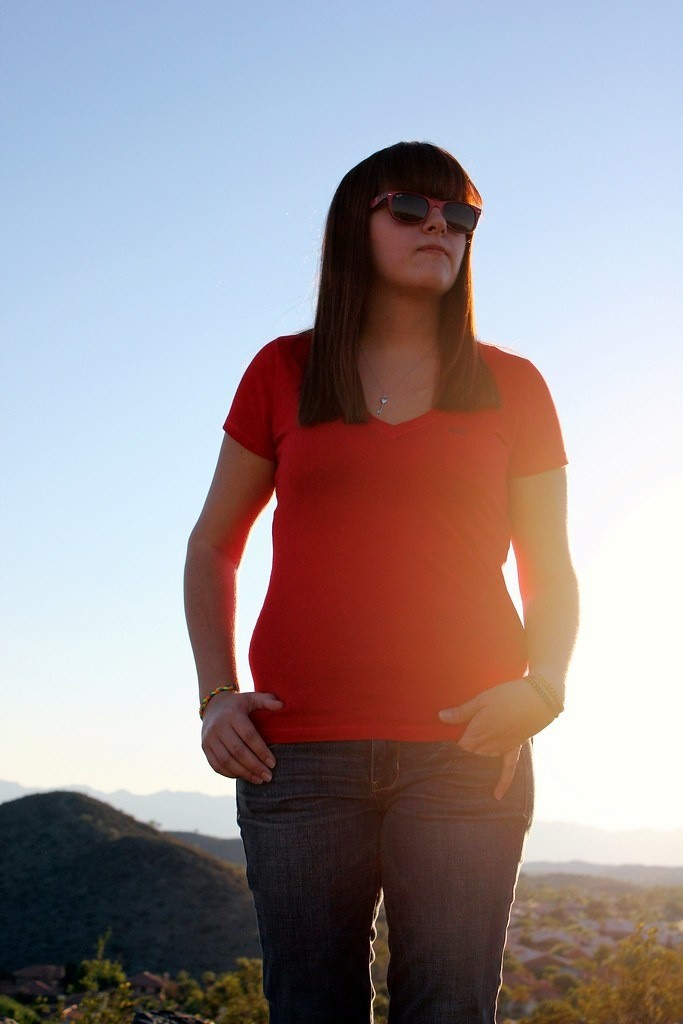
[184,142,578,1024]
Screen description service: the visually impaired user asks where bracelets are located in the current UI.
[199,684,240,720]
[523,672,564,718]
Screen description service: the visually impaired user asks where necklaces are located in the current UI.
[359,338,439,414]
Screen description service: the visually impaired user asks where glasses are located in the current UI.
[370,189,482,235]
